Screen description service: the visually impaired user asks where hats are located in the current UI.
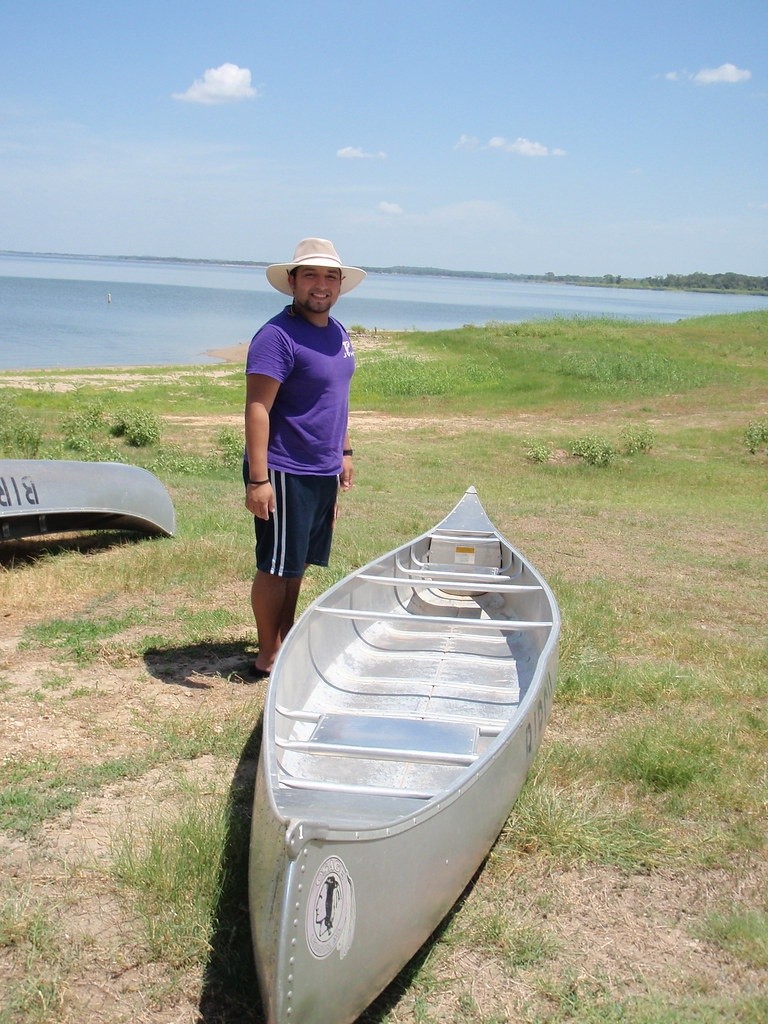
[266,238,367,297]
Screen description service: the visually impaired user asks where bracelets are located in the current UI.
[343,449,354,456]
[248,478,270,484]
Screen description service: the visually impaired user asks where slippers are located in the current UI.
[249,663,270,678]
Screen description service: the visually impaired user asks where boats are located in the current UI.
[0,460,176,537]
[248,485,561,1023]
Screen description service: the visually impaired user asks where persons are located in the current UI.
[243,239,368,676]
